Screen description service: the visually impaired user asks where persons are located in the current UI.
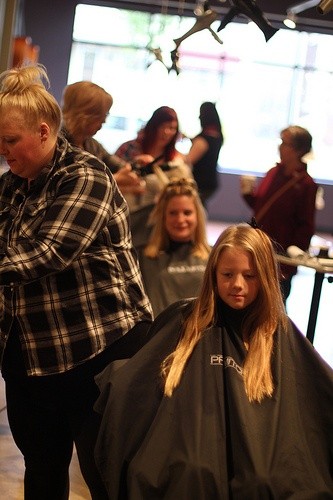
[0,68,158,500]
[240,125,318,305]
[61,81,155,195]
[94,223,333,500]
[114,107,193,210]
[134,178,214,319]
[182,102,224,203]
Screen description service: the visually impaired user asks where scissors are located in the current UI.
[133,153,172,174]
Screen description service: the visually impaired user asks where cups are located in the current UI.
[241,174,257,194]
